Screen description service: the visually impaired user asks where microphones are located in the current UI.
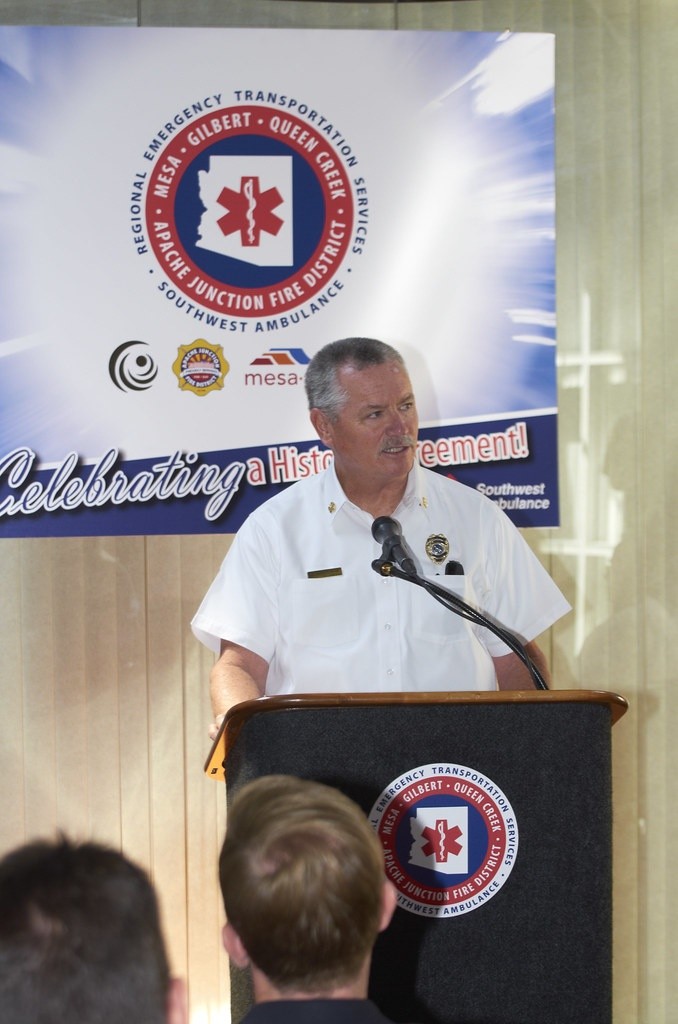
[371,516,417,577]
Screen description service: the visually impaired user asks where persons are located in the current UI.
[217,775,399,1024]
[190,337,573,742]
[0,841,190,1024]
